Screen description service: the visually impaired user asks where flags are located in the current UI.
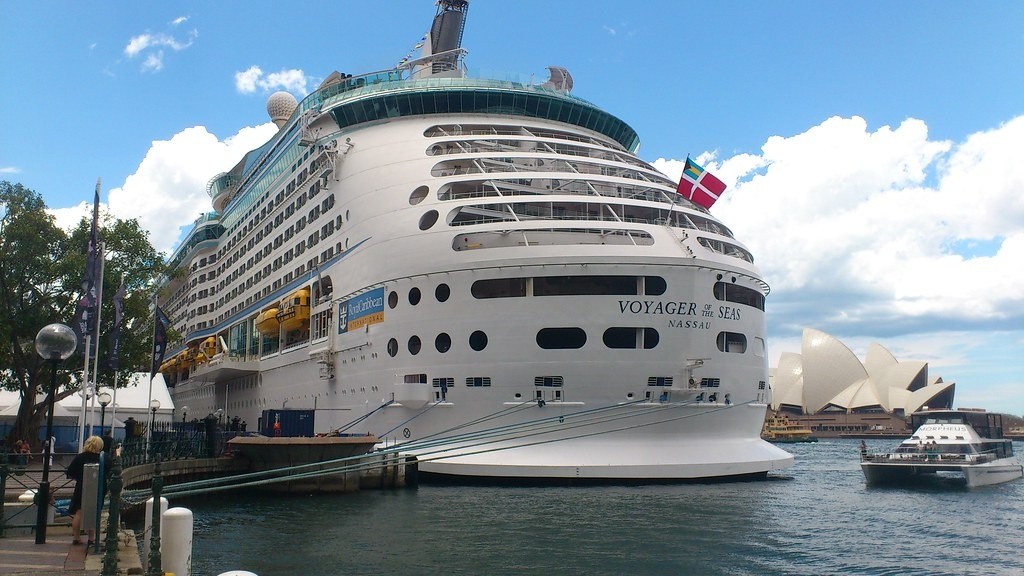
[389,34,428,78]
[69,191,102,336]
[676,157,727,209]
[108,284,125,371]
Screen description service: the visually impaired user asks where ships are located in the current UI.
[135,0,796,479]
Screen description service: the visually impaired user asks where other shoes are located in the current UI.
[49,465,52,468]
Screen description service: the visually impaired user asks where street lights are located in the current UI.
[216,409,225,426]
[35,323,78,543]
[180,405,191,422]
[150,399,161,436]
[97,392,112,437]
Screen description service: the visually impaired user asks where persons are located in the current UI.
[231,416,240,431]
[101,429,113,453]
[861,440,867,459]
[124,416,137,440]
[42,432,56,466]
[917,440,939,459]
[13,439,34,464]
[66,435,104,545]
[241,421,247,431]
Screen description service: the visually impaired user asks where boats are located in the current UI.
[254,288,310,339]
[859,409,1023,488]
[761,413,818,442]
[158,336,220,375]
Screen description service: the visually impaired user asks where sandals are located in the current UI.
[73,540,84,544]
[88,540,95,544]
